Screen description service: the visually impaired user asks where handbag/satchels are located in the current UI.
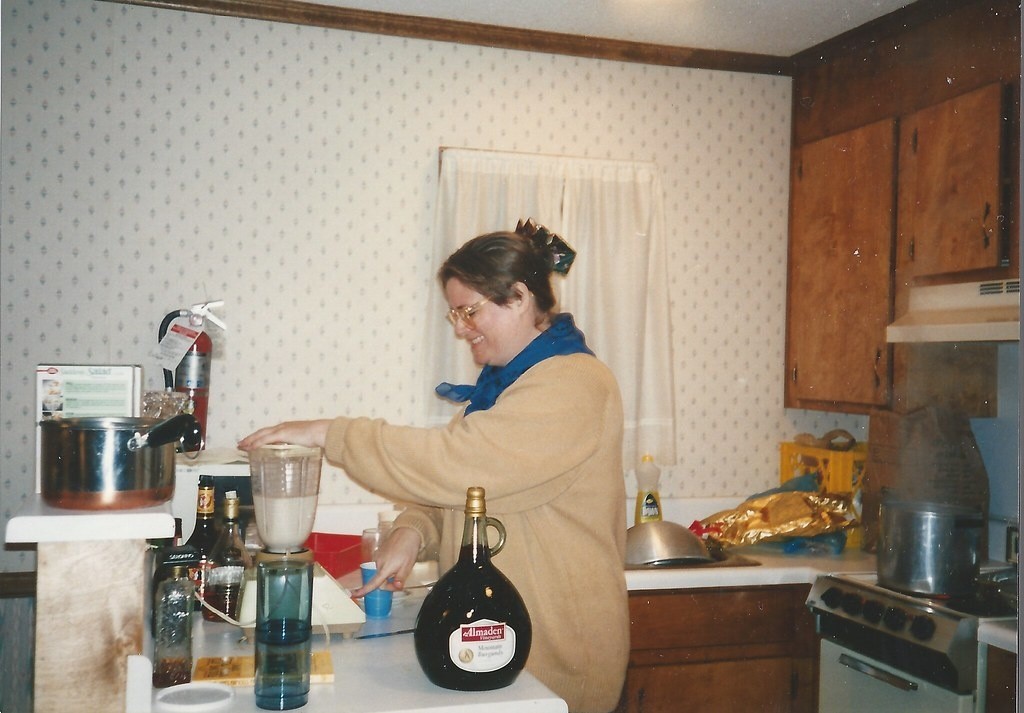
[741,471,848,556]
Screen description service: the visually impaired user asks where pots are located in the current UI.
[623,522,720,566]
[40,414,202,511]
[876,485,985,592]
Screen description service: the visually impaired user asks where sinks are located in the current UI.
[622,541,763,570]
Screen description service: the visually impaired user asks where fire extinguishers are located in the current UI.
[159,299,226,452]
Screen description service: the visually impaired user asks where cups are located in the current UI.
[360,561,394,620]
[255,558,314,710]
[144,393,191,464]
[362,529,382,562]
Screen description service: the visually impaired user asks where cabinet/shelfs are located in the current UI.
[784,0,1022,419]
[615,583,820,713]
[985,643,1018,713]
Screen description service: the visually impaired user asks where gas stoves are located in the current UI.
[804,522,1019,694]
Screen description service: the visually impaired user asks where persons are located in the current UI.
[236,219,633,713]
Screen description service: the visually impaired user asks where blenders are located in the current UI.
[240,443,366,640]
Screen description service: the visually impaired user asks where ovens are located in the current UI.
[802,606,983,712]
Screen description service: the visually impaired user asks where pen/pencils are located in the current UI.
[354,629,413,640]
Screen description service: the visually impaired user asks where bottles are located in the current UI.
[415,486,533,691]
[202,490,247,623]
[377,504,405,544]
[236,544,261,628]
[158,518,200,611]
[152,566,194,688]
[185,475,219,610]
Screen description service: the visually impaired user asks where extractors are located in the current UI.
[884,263,1022,344]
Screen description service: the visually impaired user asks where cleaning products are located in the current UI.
[634,455,663,525]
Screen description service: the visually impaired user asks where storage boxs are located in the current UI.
[302,532,376,578]
[780,440,866,548]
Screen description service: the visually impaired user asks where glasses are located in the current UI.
[447,279,533,330]
